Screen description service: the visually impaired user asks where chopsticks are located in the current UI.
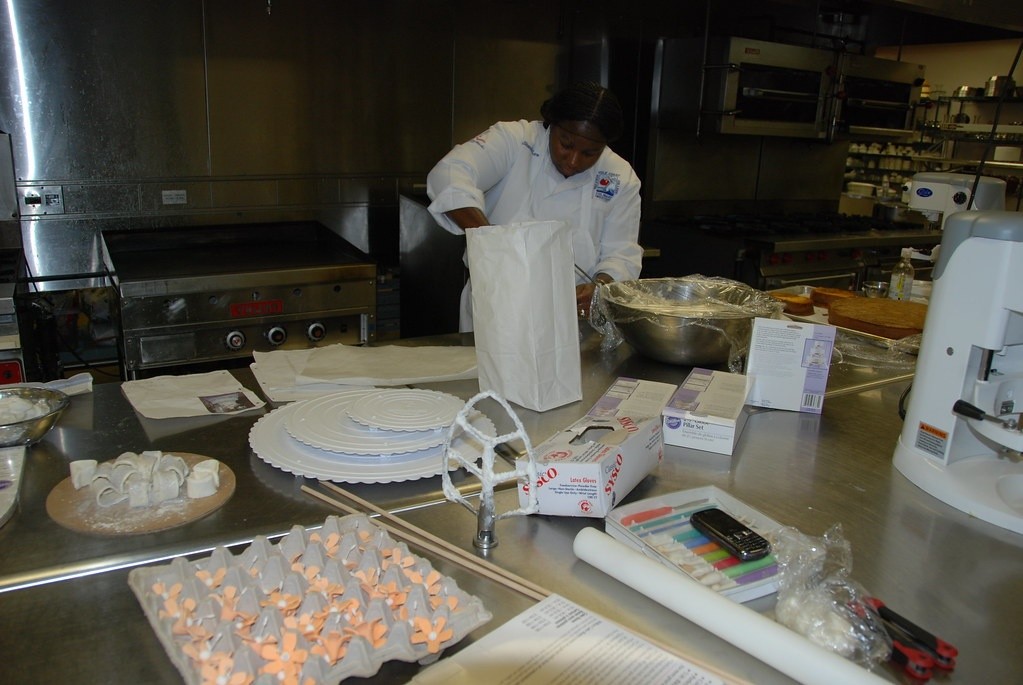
[300,480,552,602]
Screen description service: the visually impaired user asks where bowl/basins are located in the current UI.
[600,277,773,367]
[847,182,875,197]
[863,281,889,299]
[0,386,70,447]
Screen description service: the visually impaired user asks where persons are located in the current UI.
[426,86,645,334]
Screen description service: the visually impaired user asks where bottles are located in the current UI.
[887,247,914,302]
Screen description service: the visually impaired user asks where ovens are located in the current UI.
[656,37,926,146]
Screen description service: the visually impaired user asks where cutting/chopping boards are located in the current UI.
[45,452,236,536]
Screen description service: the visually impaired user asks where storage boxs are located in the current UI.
[662,368,753,455]
[516,418,662,520]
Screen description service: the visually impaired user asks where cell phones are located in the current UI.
[690,508,772,562]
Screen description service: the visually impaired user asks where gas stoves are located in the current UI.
[651,198,944,273]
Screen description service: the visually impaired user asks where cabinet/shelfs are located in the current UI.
[843,95,1023,185]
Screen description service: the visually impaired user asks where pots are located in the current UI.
[838,192,876,218]
